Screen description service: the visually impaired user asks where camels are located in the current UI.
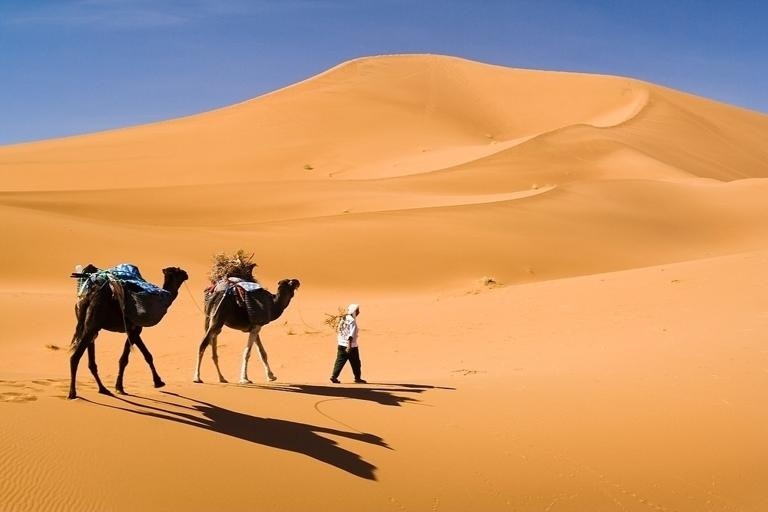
[67,264,188,399]
[193,279,301,384]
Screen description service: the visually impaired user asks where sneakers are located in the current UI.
[330,376,340,383]
[353,379,366,383]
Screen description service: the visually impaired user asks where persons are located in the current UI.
[322,303,366,384]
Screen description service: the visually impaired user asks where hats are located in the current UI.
[347,304,358,314]
